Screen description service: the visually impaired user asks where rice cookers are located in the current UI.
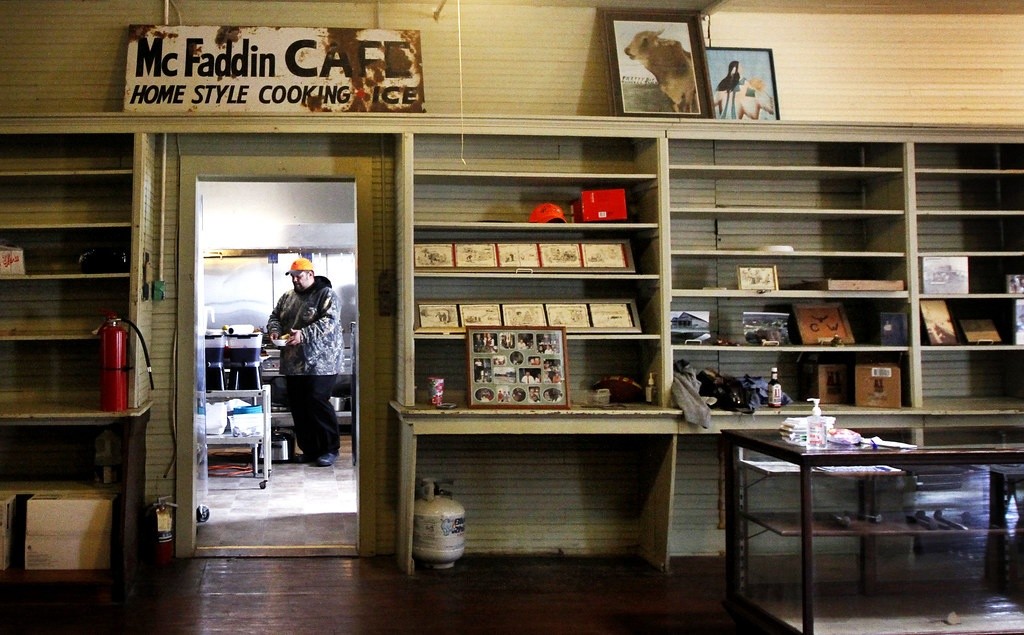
[270,431,295,463]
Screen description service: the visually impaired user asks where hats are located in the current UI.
[285,257,313,276]
[529,202,568,223]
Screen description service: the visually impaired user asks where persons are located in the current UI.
[267,257,345,467]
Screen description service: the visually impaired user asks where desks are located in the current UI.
[673,403,927,565]
[915,397,1024,447]
[721,424,1023,634]
[389,403,683,577]
[2,396,149,605]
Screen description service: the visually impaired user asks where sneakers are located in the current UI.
[317,450,341,466]
[296,453,314,465]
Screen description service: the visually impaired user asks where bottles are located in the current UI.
[807,397,827,451]
[768,367,781,408]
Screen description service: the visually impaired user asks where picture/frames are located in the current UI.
[594,5,716,121]
[706,47,782,120]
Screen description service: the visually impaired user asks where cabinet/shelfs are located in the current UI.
[399,127,1020,407]
[2,124,155,412]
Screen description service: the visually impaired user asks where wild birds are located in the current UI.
[826,321,839,332]
[811,314,829,323]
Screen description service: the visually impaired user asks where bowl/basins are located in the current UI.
[273,340,287,346]
[328,397,347,411]
